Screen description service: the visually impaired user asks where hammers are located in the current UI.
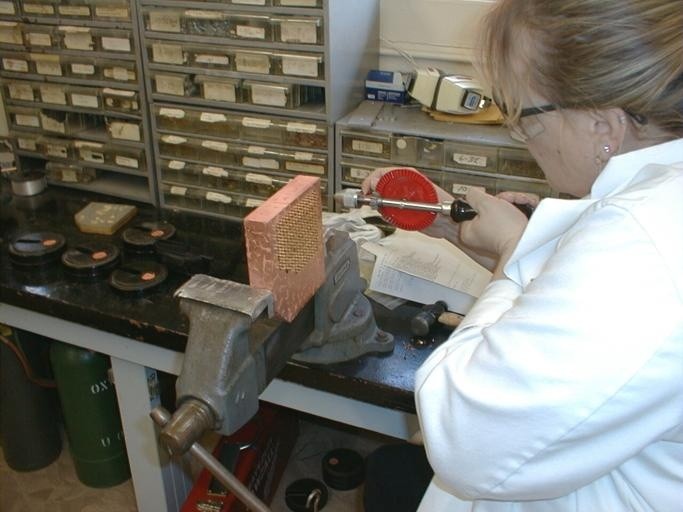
[410,301,465,337]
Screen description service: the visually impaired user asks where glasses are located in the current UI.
[490,81,580,126]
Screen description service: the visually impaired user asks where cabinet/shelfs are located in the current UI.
[136,1,381,223]
[333,97,575,227]
[1,0,159,212]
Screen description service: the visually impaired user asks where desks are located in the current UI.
[0,184,466,510]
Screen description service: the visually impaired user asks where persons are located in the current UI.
[362,0,683,512]
